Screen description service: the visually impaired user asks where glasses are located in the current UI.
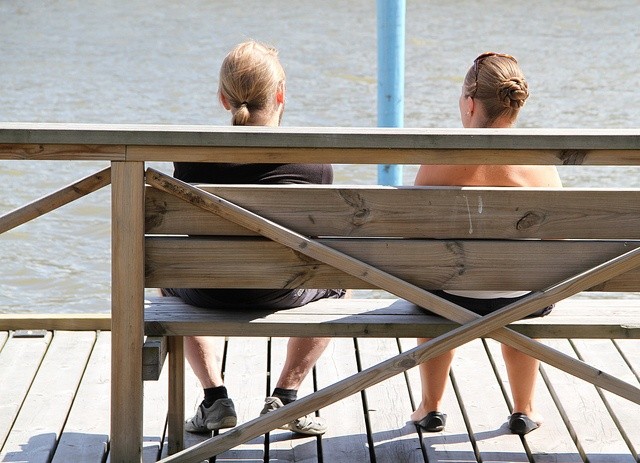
[471,52,517,100]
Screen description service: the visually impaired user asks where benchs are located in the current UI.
[135,290,640,463]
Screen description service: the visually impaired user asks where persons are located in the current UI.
[411,51,565,432]
[161,41,346,436]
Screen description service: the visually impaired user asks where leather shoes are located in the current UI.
[508,412,539,433]
[415,411,447,431]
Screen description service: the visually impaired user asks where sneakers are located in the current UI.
[184,397,237,432]
[259,395,326,434]
